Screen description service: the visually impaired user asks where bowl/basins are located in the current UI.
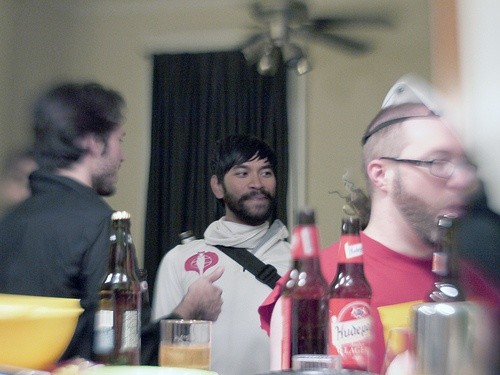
[0,293,85,372]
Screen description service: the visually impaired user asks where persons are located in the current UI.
[148,134,293,375]
[0,81,223,365]
[257,102,500,375]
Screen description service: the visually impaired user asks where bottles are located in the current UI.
[92,210,151,367]
[179,230,198,246]
[326,215,387,374]
[423,215,463,303]
[268,263,304,374]
[283,208,330,375]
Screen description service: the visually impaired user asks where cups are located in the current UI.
[414,300,499,374]
[292,353,340,375]
[158,318,213,372]
[383,328,416,373]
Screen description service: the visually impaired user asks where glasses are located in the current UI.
[378,157,480,178]
